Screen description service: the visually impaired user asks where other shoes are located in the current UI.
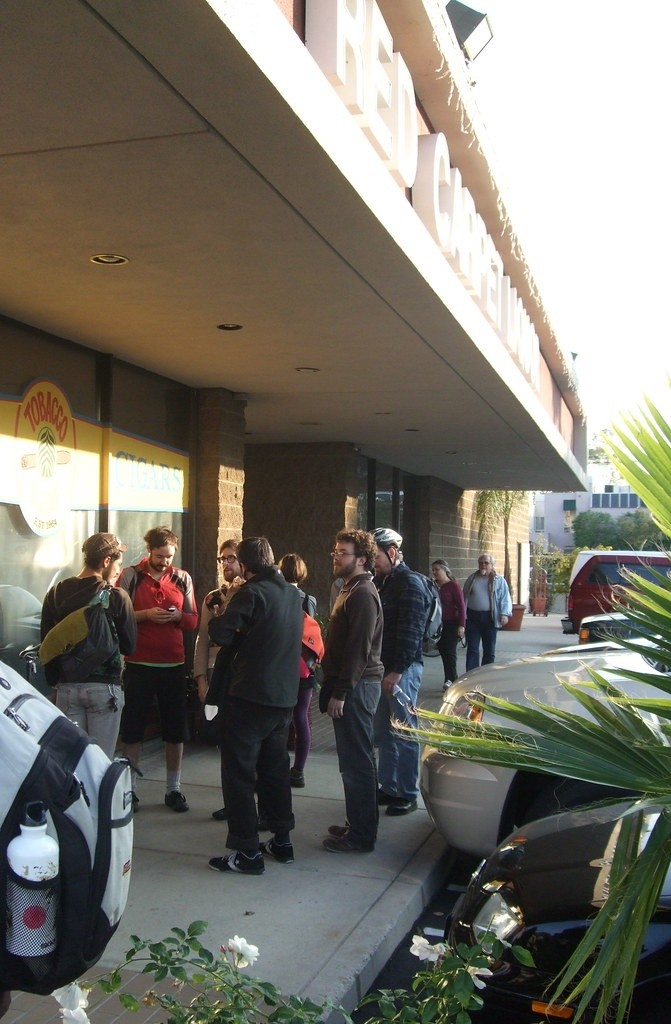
[212,808,226,820]
[289,769,305,788]
[442,680,452,692]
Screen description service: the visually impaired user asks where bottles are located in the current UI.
[5,800,57,958]
[393,684,410,707]
[211,604,222,618]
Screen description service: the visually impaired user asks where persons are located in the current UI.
[371,527,426,815]
[462,555,512,673]
[40,533,136,765]
[277,553,317,829]
[114,526,198,812]
[207,537,304,874]
[321,527,385,854]
[430,559,466,693]
[194,538,271,832]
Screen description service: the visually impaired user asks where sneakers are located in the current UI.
[260,837,294,862]
[323,837,376,853]
[208,851,265,874]
[165,790,189,812]
[132,792,139,813]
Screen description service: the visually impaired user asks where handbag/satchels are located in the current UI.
[37,602,119,684]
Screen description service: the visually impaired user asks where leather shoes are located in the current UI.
[378,790,394,805]
[386,799,418,815]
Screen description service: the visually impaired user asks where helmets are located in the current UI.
[369,527,403,548]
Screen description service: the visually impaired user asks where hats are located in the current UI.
[82,531,123,559]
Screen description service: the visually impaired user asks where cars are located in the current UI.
[568,554,671,632]
[0,585,44,642]
[560,550,671,634]
[444,797,671,1024]
[419,634,671,859]
[579,610,658,646]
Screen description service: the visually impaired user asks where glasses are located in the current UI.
[216,555,238,564]
[330,551,355,557]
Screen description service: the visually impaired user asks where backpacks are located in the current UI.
[0,658,134,995]
[299,589,324,682]
[393,566,443,654]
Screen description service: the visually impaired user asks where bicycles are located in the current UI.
[422,625,467,658]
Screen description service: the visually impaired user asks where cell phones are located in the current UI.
[168,608,176,611]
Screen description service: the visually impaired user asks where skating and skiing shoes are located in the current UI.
[328,825,349,835]
